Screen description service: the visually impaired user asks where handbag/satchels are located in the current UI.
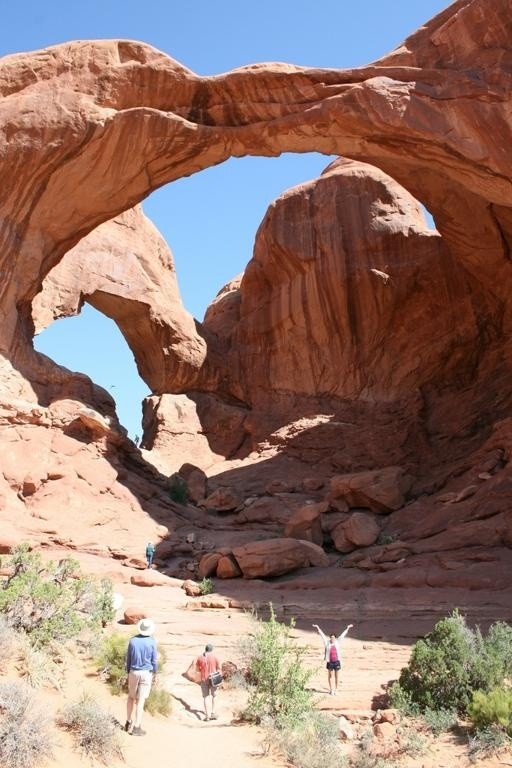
[208,672,223,687]
[181,659,202,685]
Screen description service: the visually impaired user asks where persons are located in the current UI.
[121,618,159,737]
[145,541,155,569]
[196,644,222,721]
[311,623,354,697]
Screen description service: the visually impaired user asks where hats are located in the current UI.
[139,619,155,636]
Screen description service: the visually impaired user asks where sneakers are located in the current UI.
[335,690,342,695]
[132,726,146,735]
[329,689,334,695]
[125,720,132,731]
[204,716,209,720]
[210,713,218,719]
